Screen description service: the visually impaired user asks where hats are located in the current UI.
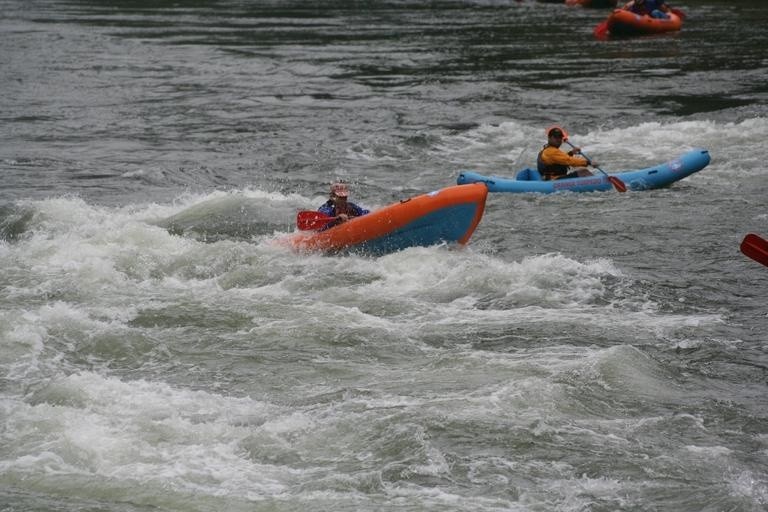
[329,183,348,197]
[549,128,563,137]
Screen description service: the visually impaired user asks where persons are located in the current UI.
[538,128,598,181]
[318,183,369,232]
[623,0,670,18]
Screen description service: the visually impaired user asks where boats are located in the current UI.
[608,0,681,34]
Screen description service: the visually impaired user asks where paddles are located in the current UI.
[663,2,687,19]
[297,211,357,230]
[595,0,633,38]
[546,124,627,193]
[740,232,768,267]
